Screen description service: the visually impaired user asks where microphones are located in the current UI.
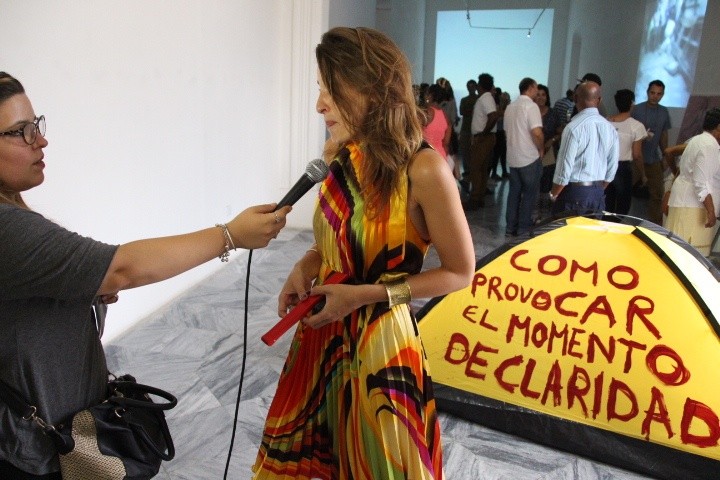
[272,158,328,211]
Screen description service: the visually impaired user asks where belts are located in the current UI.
[570,180,605,187]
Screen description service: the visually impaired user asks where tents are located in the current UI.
[412,211,720,480]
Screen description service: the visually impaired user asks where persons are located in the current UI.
[404,72,720,259]
[0,72,294,480]
[253,26,476,480]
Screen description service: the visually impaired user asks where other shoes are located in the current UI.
[505,225,524,235]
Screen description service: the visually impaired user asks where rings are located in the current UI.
[274,211,281,223]
[273,231,277,239]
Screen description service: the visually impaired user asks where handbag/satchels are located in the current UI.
[49,374,178,480]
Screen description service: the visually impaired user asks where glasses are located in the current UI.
[0,114,47,145]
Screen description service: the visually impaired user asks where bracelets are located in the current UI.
[215,224,237,264]
[551,137,556,143]
[549,191,558,200]
[306,249,321,258]
[375,272,413,309]
